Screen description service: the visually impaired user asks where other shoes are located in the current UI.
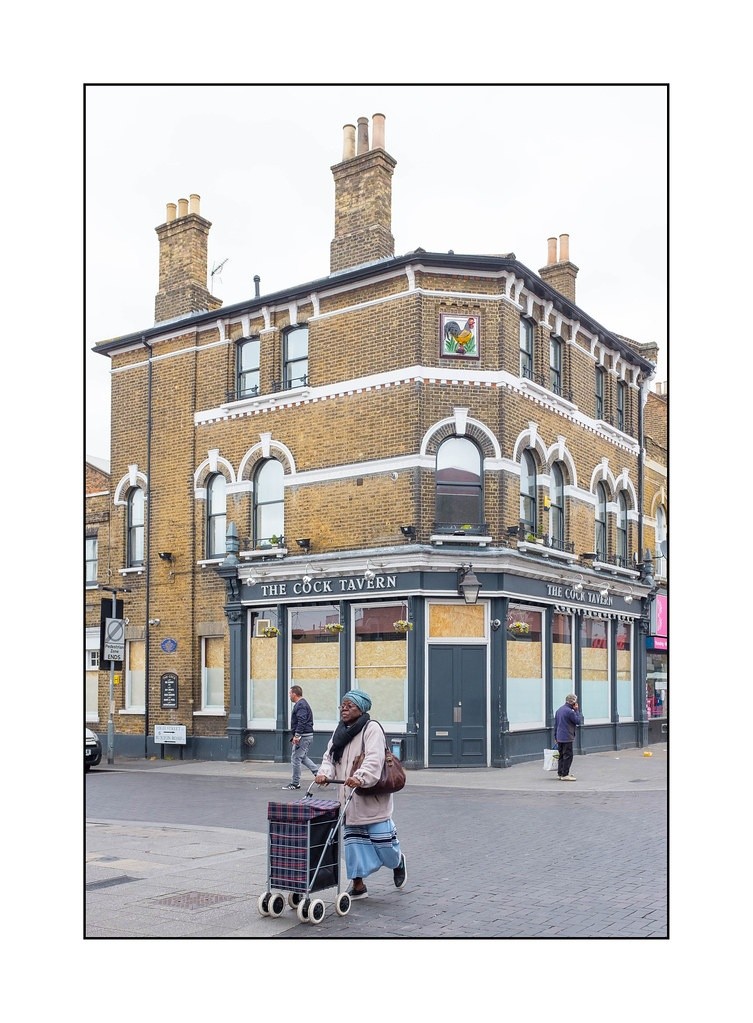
[558,774,577,781]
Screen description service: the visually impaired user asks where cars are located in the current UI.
[86,728,102,774]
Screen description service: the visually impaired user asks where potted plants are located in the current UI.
[270,535,278,549]
[536,524,545,547]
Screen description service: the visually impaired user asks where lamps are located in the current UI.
[458,562,483,604]
[580,552,597,559]
[400,526,415,537]
[158,553,172,560]
[617,586,633,605]
[593,582,610,598]
[571,574,584,593]
[295,539,309,548]
[507,526,518,534]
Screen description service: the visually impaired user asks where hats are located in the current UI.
[566,694,577,705]
[342,691,372,713]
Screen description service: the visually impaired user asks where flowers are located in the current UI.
[262,627,280,637]
[509,622,529,634]
[393,620,414,633]
[325,622,344,632]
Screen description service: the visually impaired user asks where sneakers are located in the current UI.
[347,884,369,900]
[282,782,301,790]
[393,853,407,888]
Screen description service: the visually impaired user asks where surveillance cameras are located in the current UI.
[494,620,500,626]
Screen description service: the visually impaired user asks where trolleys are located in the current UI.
[257,779,358,925]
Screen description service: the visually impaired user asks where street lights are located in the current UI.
[97,586,132,766]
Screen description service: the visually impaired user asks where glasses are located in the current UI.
[338,704,358,711]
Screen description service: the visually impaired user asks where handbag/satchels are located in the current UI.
[543,742,559,771]
[350,720,406,796]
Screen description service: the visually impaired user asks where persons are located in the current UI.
[553,694,580,781]
[282,686,330,790]
[315,689,408,901]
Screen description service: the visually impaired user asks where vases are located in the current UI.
[328,627,340,632]
[398,626,408,633]
[264,632,276,637]
[513,628,525,633]
